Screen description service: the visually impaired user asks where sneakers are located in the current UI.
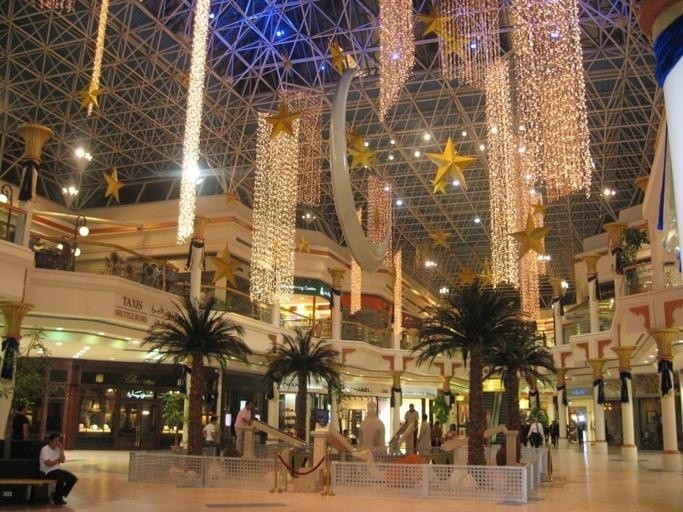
[52,496,67,506]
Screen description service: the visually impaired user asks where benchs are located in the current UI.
[0,475,58,505]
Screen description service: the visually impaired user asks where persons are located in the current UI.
[430,421,442,464]
[416,414,431,456]
[234,402,253,451]
[445,422,457,440]
[202,416,218,447]
[520,417,560,448]
[39,432,80,506]
[403,404,420,451]
[210,414,221,444]
[576,419,587,446]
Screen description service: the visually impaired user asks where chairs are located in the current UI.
[428,444,502,484]
